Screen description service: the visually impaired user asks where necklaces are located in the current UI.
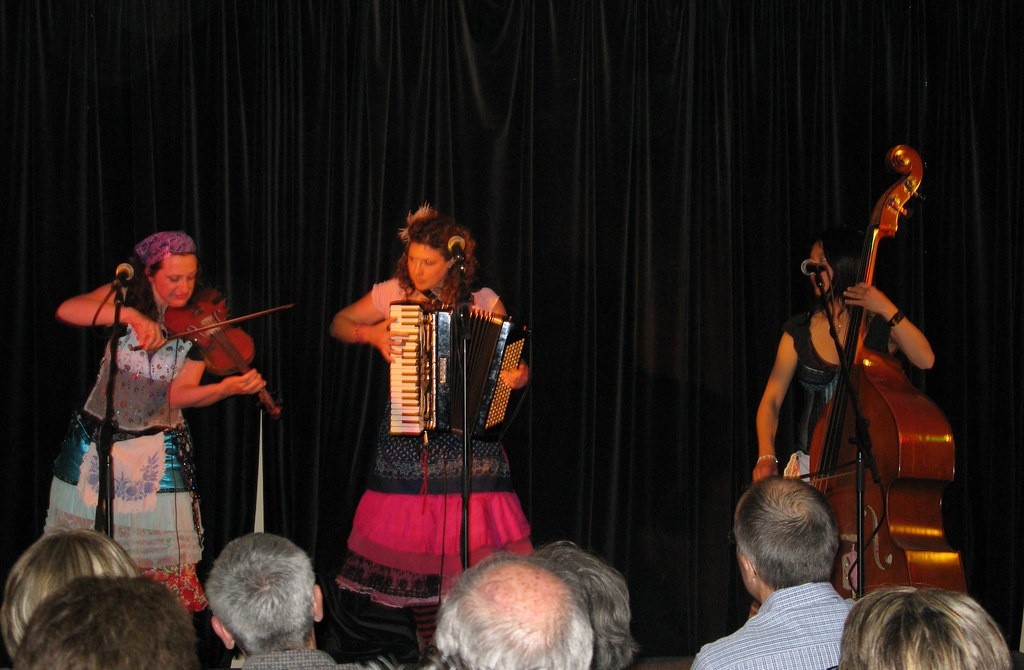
[835,323,842,334]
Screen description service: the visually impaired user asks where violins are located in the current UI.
[163,286,284,422]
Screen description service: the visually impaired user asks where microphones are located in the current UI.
[114,262,135,287]
[448,235,465,263]
[801,259,828,276]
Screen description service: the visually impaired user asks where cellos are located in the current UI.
[809,143,969,603]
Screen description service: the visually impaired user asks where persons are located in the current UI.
[330,208,533,648]
[753,225,935,484]
[535,541,635,670]
[689,474,857,670]
[0,528,142,662]
[42,231,267,618]
[837,585,1013,670]
[205,532,355,670]
[435,552,595,670]
[13,576,202,670]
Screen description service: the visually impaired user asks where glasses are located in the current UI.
[811,257,828,265]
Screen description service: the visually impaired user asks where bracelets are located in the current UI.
[757,455,778,463]
[888,310,904,327]
[354,323,365,344]
